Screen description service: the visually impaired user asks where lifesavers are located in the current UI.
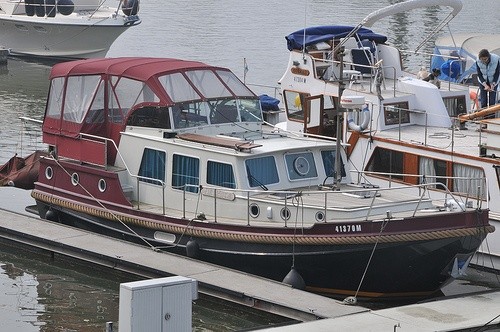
[122,0,140,16]
[56,0,74,16]
[470,91,479,110]
[25,0,55,17]
[347,104,370,131]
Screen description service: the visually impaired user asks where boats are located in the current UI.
[0,0,142,66]
[13,57,497,305]
[270,2,500,257]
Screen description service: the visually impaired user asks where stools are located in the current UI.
[347,74,364,92]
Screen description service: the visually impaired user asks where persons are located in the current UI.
[476,49,500,129]
